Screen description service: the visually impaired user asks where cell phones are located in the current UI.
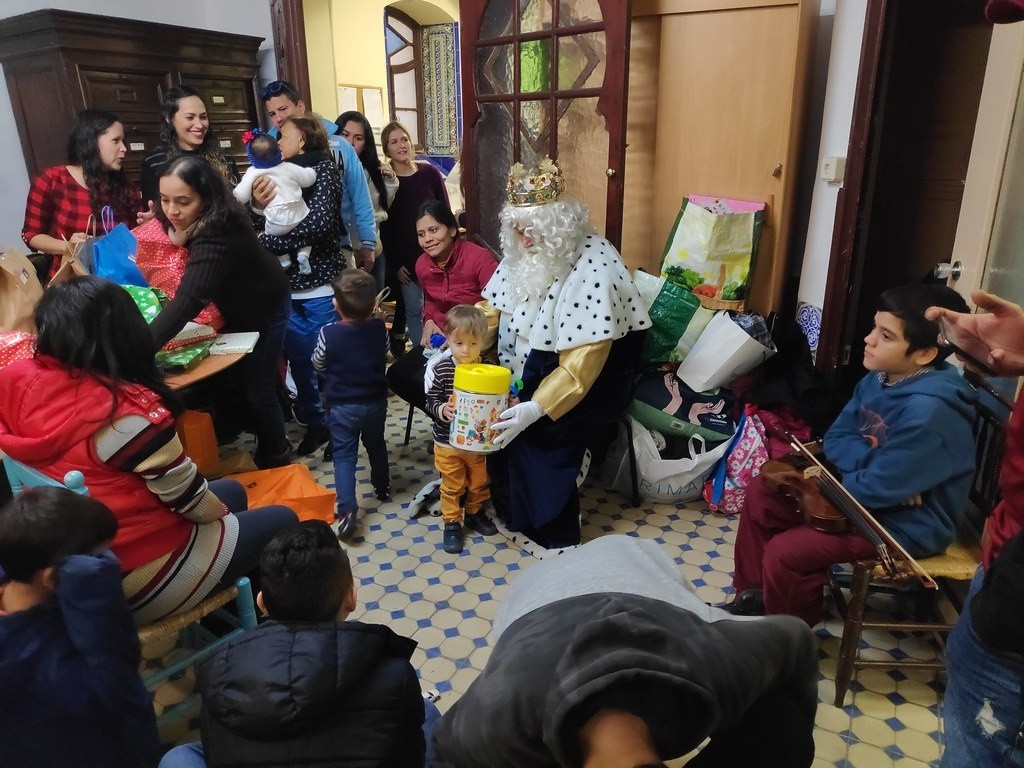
[936,314,1000,378]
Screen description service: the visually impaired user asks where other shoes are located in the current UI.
[323,443,333,462]
[297,424,329,457]
[254,437,305,468]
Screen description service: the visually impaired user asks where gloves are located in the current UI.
[490,400,542,448]
[423,349,454,394]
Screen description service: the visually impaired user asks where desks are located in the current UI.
[165,333,249,391]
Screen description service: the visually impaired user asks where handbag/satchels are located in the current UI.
[220,463,335,527]
[603,196,811,515]
[1,204,222,372]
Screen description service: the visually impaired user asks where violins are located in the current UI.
[759,453,919,593]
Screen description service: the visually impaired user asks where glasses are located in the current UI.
[260,82,300,101]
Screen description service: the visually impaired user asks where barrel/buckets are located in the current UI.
[448,364,511,454]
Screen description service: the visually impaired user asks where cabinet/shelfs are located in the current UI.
[0,6,266,183]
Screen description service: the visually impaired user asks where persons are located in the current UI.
[259,80,377,270]
[0,487,159,768]
[247,117,348,458]
[20,109,145,278]
[381,121,451,348]
[431,534,818,767]
[140,87,244,221]
[139,155,293,470]
[234,128,317,275]
[311,267,389,537]
[923,287,1024,768]
[716,280,981,614]
[425,305,519,553]
[385,198,503,454]
[0,243,45,368]
[334,110,400,299]
[0,278,299,640]
[385,268,407,359]
[159,519,443,768]
[408,159,652,559]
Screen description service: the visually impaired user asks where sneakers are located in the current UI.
[443,523,462,552]
[339,510,356,535]
[371,475,391,500]
[464,509,498,535]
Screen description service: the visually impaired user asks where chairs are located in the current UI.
[2,452,260,726]
[824,365,1015,707]
